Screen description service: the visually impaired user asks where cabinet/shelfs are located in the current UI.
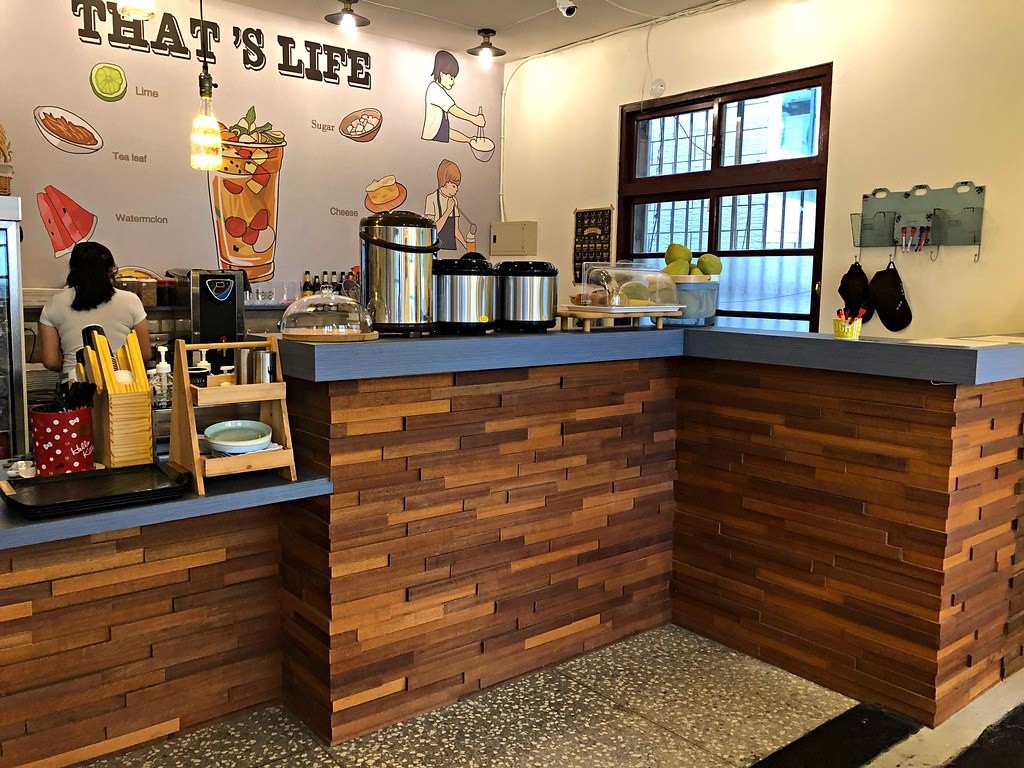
[169,335,298,496]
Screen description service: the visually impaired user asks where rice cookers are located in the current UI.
[432,251,499,333]
[496,260,559,333]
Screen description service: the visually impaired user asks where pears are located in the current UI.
[620,281,650,301]
[661,243,723,276]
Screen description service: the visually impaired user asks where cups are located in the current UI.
[234,348,255,384]
[188,367,208,388]
[253,351,276,384]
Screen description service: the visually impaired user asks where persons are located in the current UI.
[40,242,151,395]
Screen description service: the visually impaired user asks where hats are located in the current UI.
[866,262,912,332]
[837,262,875,325]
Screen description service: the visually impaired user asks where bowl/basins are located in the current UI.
[833,317,863,340]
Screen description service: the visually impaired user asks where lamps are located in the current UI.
[466,28,508,71]
[189,0,222,170]
[324,0,371,39]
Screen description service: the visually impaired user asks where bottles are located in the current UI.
[321,271,330,294]
[348,272,353,281]
[314,276,321,296]
[303,270,314,297]
[338,272,346,295]
[330,271,339,295]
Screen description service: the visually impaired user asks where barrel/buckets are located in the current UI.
[649,275,719,327]
[359,209,442,335]
[30,405,95,475]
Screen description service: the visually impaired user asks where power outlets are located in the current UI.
[23,321,39,335]
[147,318,192,333]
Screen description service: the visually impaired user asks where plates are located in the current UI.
[203,420,272,453]
